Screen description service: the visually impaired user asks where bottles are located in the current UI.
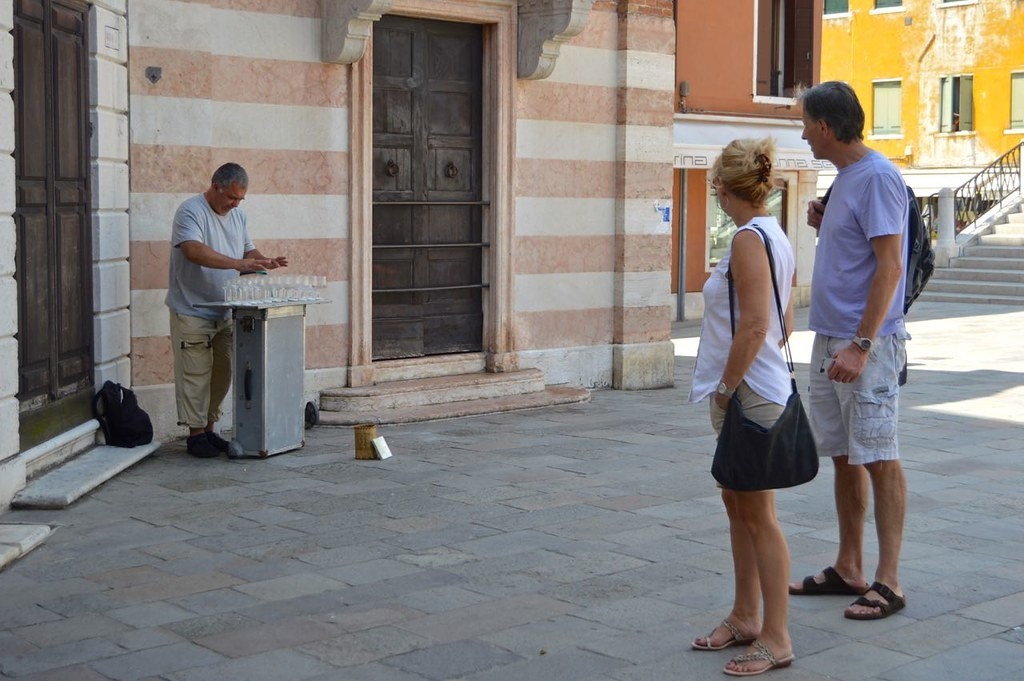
[224,272,328,304]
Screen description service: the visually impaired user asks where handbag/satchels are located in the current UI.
[711,393,819,491]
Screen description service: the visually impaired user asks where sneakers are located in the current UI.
[187,431,230,458]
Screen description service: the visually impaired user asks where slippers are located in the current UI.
[844,582,906,620]
[788,566,870,596]
[723,640,794,675]
[691,612,757,651]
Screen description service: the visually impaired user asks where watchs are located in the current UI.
[853,337,875,352]
[716,381,736,398]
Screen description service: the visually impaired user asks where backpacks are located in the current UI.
[815,186,935,314]
[91,380,153,448]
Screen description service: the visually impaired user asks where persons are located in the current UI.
[689,138,798,676]
[164,162,290,457]
[786,81,909,620]
